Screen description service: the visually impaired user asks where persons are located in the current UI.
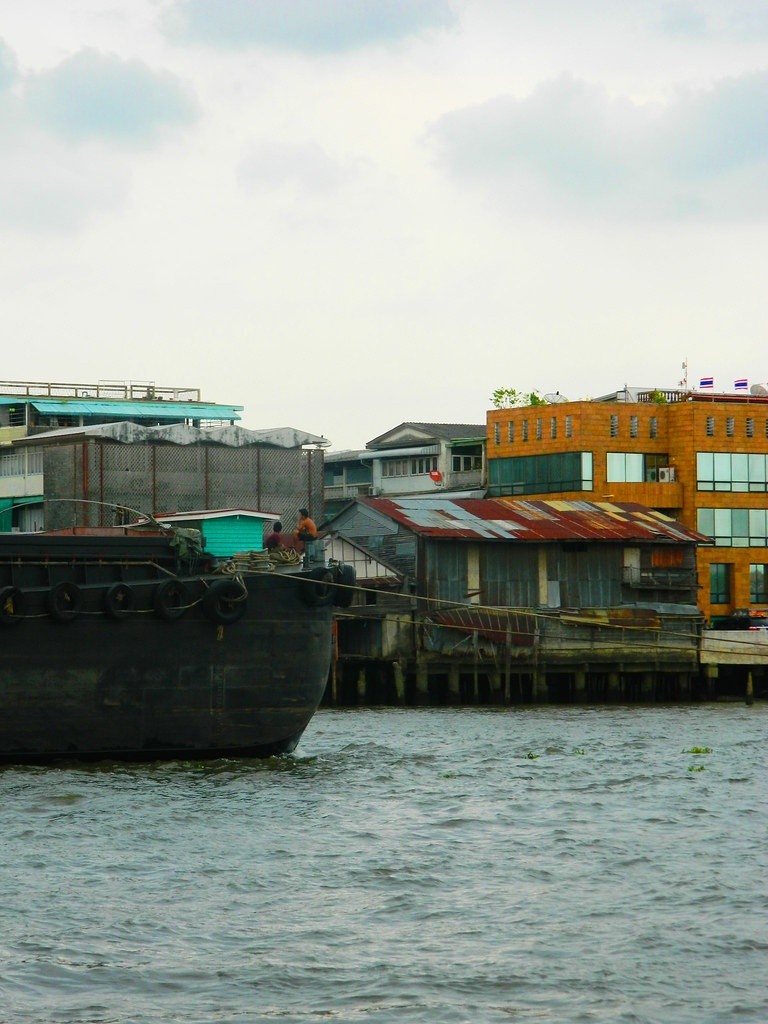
[265,522,287,553]
[293,509,318,554]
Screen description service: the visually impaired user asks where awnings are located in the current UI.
[32,403,242,420]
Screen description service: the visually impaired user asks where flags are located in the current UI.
[734,379,747,390]
[700,377,713,388]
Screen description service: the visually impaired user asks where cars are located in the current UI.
[712,608,768,632]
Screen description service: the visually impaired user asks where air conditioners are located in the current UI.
[659,468,675,482]
[646,469,657,482]
[368,487,378,496]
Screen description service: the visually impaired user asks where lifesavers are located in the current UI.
[332,564,357,609]
[154,579,191,622]
[204,579,248,626]
[1,586,27,627]
[104,583,137,622]
[47,582,85,623]
[305,567,337,608]
